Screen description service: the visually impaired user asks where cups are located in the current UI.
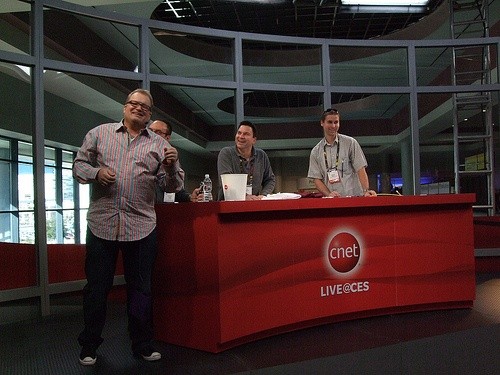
[245,184,252,198]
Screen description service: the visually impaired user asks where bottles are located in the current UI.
[202,174,213,202]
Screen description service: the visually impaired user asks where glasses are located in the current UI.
[322,109,338,116]
[149,128,168,135]
[126,100,151,111]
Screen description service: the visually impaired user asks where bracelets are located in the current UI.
[363,189,368,192]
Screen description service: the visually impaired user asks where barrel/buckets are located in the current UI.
[219,173,248,201]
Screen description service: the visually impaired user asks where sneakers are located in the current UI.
[79,348,97,365]
[132,345,163,360]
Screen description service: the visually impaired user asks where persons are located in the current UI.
[217,121,276,202]
[307,109,376,197]
[72,88,184,364]
[149,119,204,203]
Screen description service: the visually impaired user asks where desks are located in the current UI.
[148,193,477,353]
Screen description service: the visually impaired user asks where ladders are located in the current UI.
[450,0,495,216]
